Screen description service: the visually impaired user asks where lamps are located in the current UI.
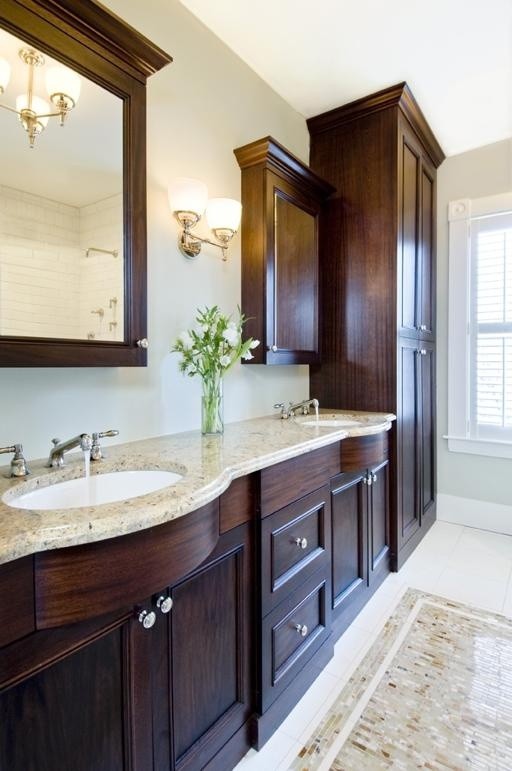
[0,29,83,151]
[171,172,243,263]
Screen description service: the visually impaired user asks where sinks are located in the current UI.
[294,413,369,427]
[2,463,187,510]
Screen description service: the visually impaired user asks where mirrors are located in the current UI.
[0,1,172,369]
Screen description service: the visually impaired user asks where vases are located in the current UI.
[200,376,225,436]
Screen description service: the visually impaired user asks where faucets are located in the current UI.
[291,399,319,413]
[46,433,93,466]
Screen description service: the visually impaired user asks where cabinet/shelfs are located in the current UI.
[234,135,338,366]
[306,79,448,575]
[3,529,250,770]
[327,459,395,623]
[260,484,333,717]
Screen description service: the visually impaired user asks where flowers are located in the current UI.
[168,299,262,431]
